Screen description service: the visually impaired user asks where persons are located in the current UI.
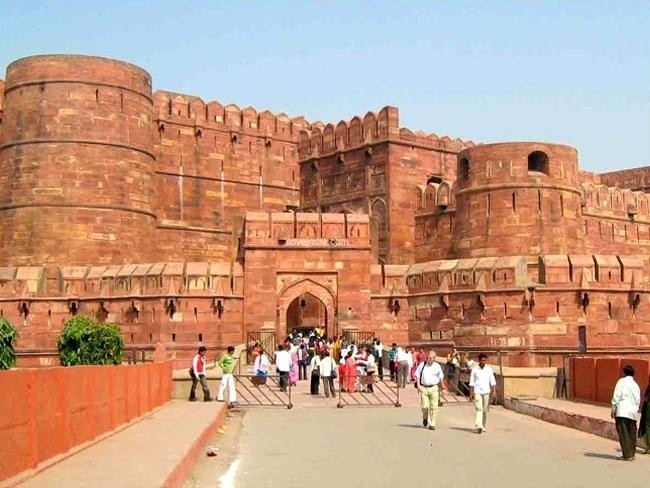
[609,365,642,461]
[190,346,214,401]
[217,345,240,404]
[635,375,650,453]
[414,351,447,430]
[469,352,499,432]
[249,330,456,406]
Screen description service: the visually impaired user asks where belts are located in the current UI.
[423,385,434,387]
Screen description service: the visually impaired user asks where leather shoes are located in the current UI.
[424,419,427,426]
[429,426,435,430]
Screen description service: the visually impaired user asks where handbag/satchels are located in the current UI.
[414,381,422,388]
[332,371,337,378]
[256,369,267,378]
[312,369,320,377]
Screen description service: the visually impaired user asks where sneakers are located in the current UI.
[616,456,632,460]
